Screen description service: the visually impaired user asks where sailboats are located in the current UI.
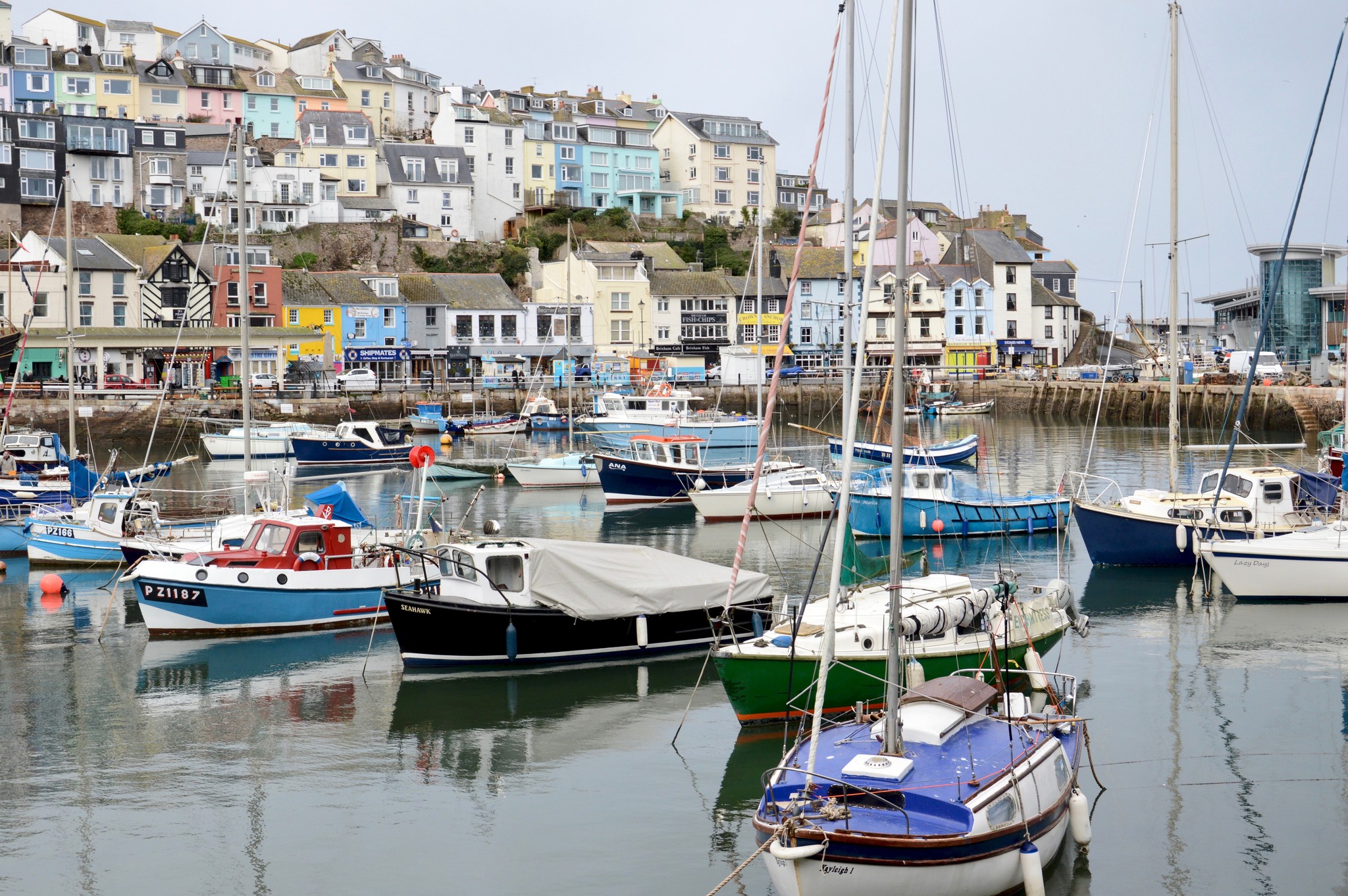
[2,111,774,686]
[696,0,1348,896]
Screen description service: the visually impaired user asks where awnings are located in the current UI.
[1000,346,1036,356]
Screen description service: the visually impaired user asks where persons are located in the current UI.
[0,451,17,478]
[20,367,527,402]
[126,502,144,537]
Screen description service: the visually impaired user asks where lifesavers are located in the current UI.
[658,383,671,397]
[417,401,427,403]
[647,390,660,397]
[529,397,537,402]
[293,551,326,571]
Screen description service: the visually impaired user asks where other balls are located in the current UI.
[932,519,944,532]
[695,478,706,490]
[1263,378,1272,386]
[39,574,66,594]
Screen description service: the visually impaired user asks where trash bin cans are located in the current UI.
[228,379,240,393]
[420,371,434,389]
[220,375,240,392]
